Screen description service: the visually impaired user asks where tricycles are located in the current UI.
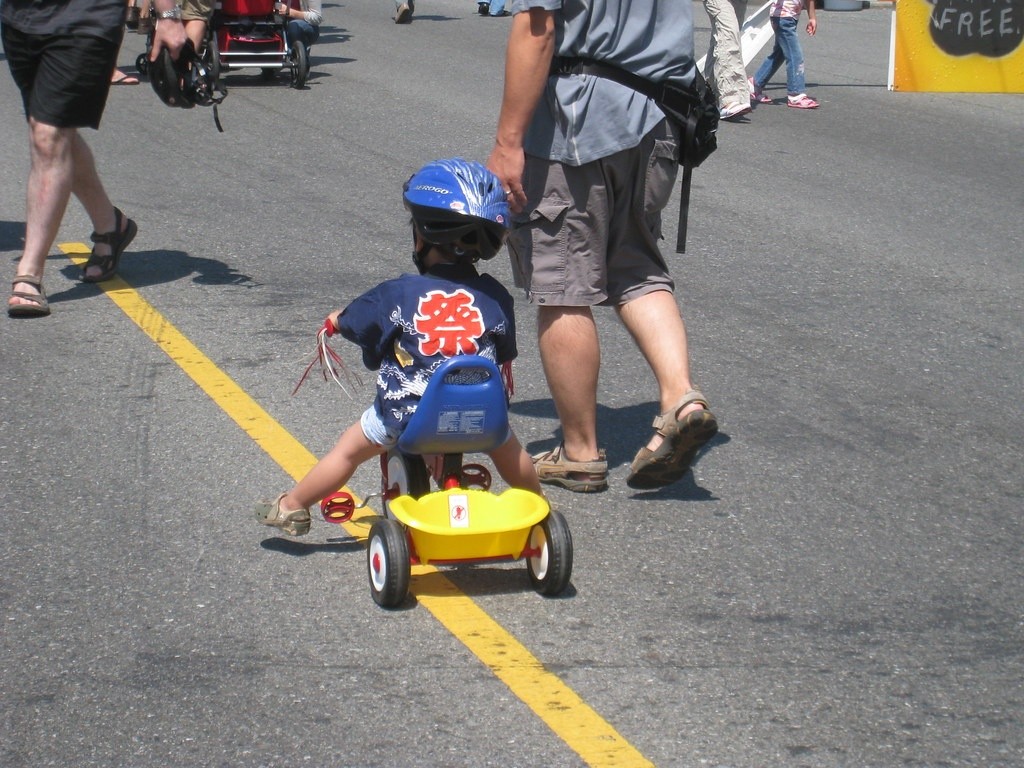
[321,319,573,609]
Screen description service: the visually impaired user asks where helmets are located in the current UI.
[146,39,199,109]
[402,157,510,262]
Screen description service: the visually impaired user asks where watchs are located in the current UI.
[157,7,182,22]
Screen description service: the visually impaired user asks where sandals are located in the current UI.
[530,439,608,493]
[625,392,718,489]
[255,492,312,537]
[6,275,51,315]
[788,93,819,108]
[78,206,138,282]
[747,79,771,102]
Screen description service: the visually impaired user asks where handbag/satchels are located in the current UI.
[546,57,720,168]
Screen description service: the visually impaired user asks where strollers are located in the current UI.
[203,0,307,90]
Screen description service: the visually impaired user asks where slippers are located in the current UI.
[110,76,140,85]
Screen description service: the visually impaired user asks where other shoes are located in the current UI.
[719,101,751,120]
[396,2,416,25]
[491,10,509,17]
[478,3,489,15]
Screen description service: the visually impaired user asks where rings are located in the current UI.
[506,191,512,195]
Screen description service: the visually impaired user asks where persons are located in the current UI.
[483,0,720,494]
[0,0,139,315]
[273,0,323,79]
[111,0,217,87]
[477,0,507,17]
[392,0,416,24]
[255,162,548,536]
[703,0,752,119]
[745,0,820,110]
